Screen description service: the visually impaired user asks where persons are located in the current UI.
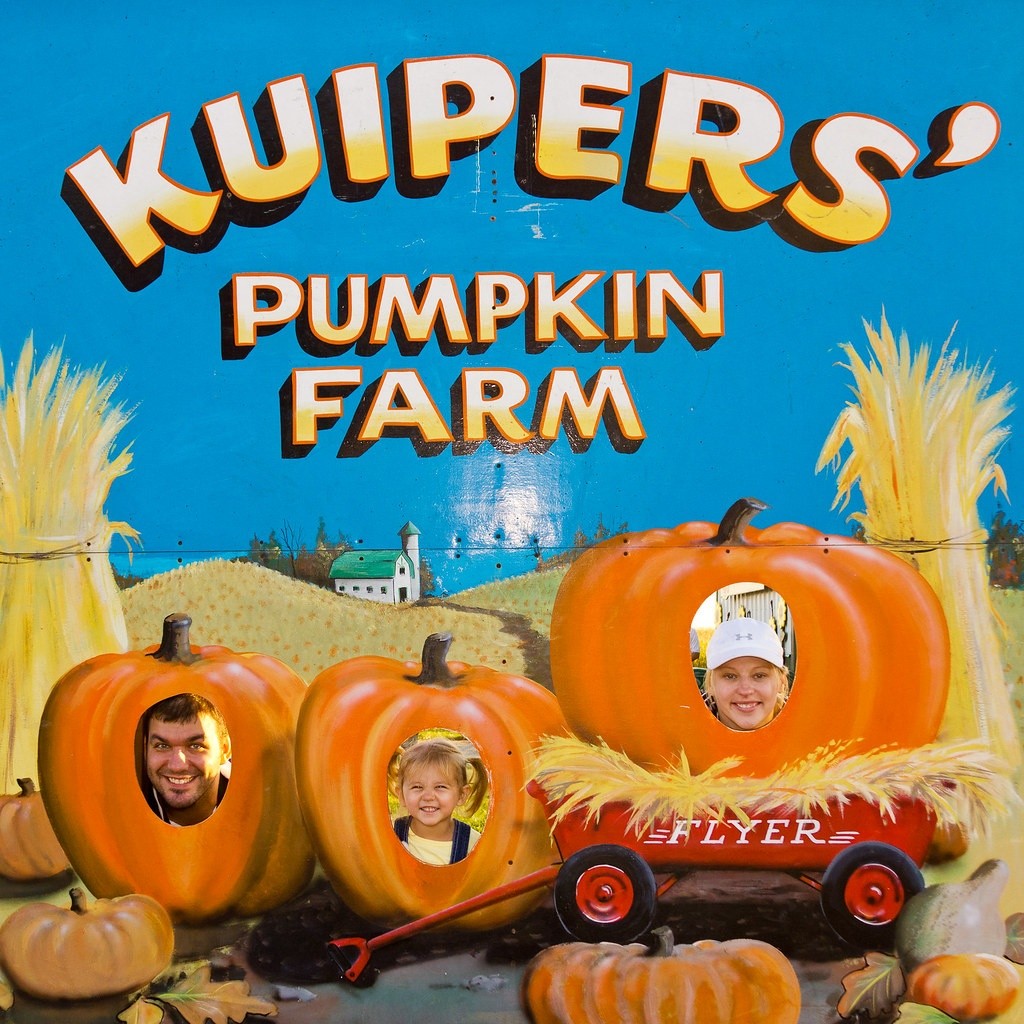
[135,695,229,827]
[706,617,787,731]
[386,737,489,868]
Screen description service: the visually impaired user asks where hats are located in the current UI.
[706,617,783,672]
